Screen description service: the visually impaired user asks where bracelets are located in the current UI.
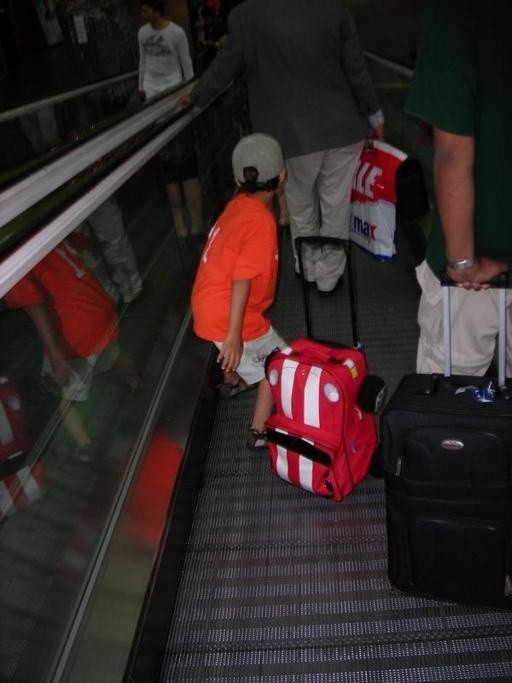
[447,253,478,271]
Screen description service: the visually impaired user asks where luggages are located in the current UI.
[0,372,51,518]
[378,272,512,608]
[261,234,381,501]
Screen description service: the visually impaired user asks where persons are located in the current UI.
[193,1,236,77]
[5,238,122,464]
[189,130,290,452]
[135,0,196,103]
[160,127,209,248]
[71,195,144,308]
[403,2,512,386]
[174,1,387,296]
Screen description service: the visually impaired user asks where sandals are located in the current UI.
[245,426,268,451]
[220,376,258,399]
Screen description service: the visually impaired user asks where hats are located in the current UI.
[231,133,284,185]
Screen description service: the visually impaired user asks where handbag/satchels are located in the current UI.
[348,138,409,262]
